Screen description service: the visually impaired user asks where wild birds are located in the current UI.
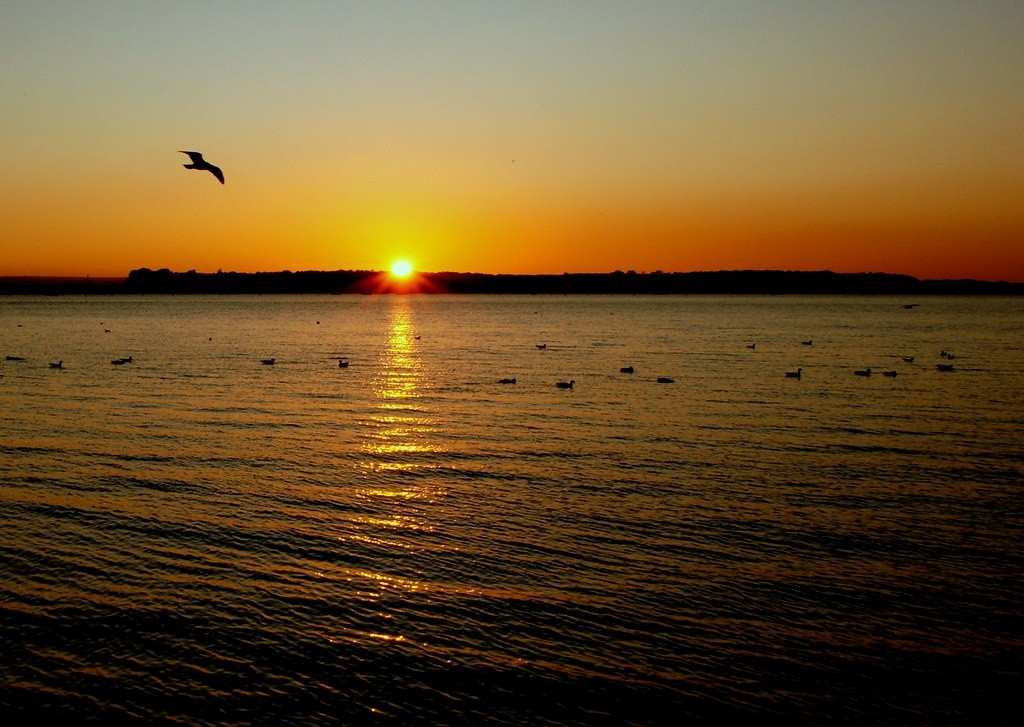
[338,359,350,368]
[656,377,675,384]
[535,344,547,348]
[853,368,872,378]
[936,363,954,371]
[415,335,421,339]
[497,378,517,384]
[619,366,635,374]
[317,320,320,324]
[801,340,813,346]
[104,328,111,332]
[49,360,64,368]
[747,343,755,349]
[178,150,225,185]
[902,356,914,362]
[555,379,576,388]
[784,368,803,377]
[939,349,955,361]
[882,370,897,377]
[111,356,133,365]
[260,358,276,365]
